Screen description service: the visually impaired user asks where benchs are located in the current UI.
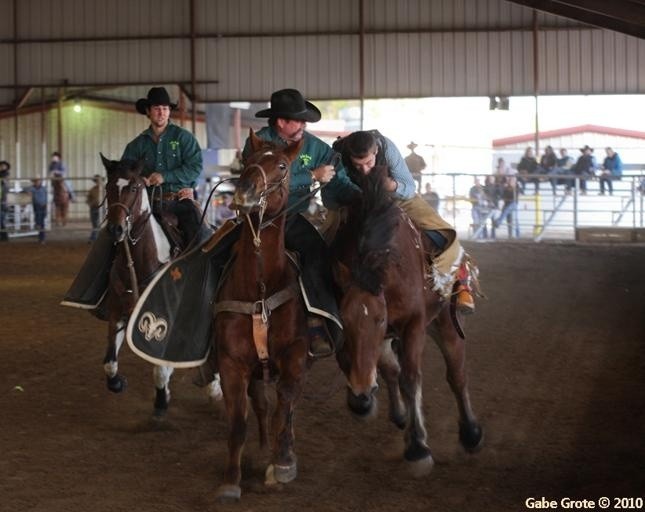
[471,176,638,243]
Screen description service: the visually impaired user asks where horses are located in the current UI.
[207,127,311,505]
[328,166,487,481]
[96,150,224,422]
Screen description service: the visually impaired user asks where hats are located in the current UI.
[256,89,321,122]
[580,145,593,153]
[136,87,177,114]
[407,141,417,148]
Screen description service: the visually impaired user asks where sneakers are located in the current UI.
[457,285,475,313]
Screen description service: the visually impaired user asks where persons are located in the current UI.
[87,175,107,244]
[320,130,475,315]
[48,152,78,203]
[24,174,48,242]
[121,87,207,253]
[470,145,623,240]
[242,88,363,359]
[422,183,439,214]
[0,161,10,241]
[404,141,426,194]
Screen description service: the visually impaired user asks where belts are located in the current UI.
[150,192,177,201]
